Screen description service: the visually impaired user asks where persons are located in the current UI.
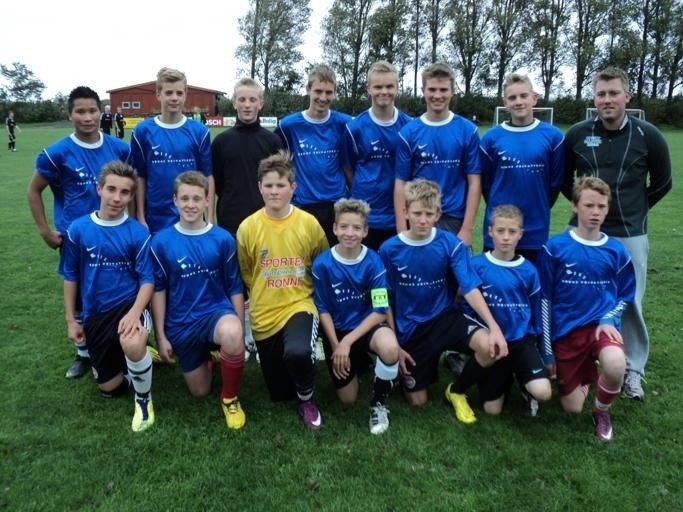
[398,64,483,378]
[342,61,416,366]
[560,66,673,400]
[377,179,508,423]
[114,106,125,139]
[478,73,566,380]
[5,110,21,152]
[310,197,399,435]
[274,63,353,361]
[212,80,283,363]
[151,171,245,430]
[201,113,207,124]
[132,68,221,309]
[236,149,330,430]
[100,105,113,136]
[27,85,131,379]
[535,176,636,440]
[58,159,168,431]
[462,203,552,419]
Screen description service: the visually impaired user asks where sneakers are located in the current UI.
[146,345,163,364]
[366,404,393,436]
[295,399,322,430]
[130,391,156,433]
[591,405,615,443]
[218,394,248,430]
[63,354,93,380]
[443,353,467,376]
[209,350,222,362]
[315,337,327,360]
[519,390,540,418]
[444,382,477,425]
[616,369,649,403]
